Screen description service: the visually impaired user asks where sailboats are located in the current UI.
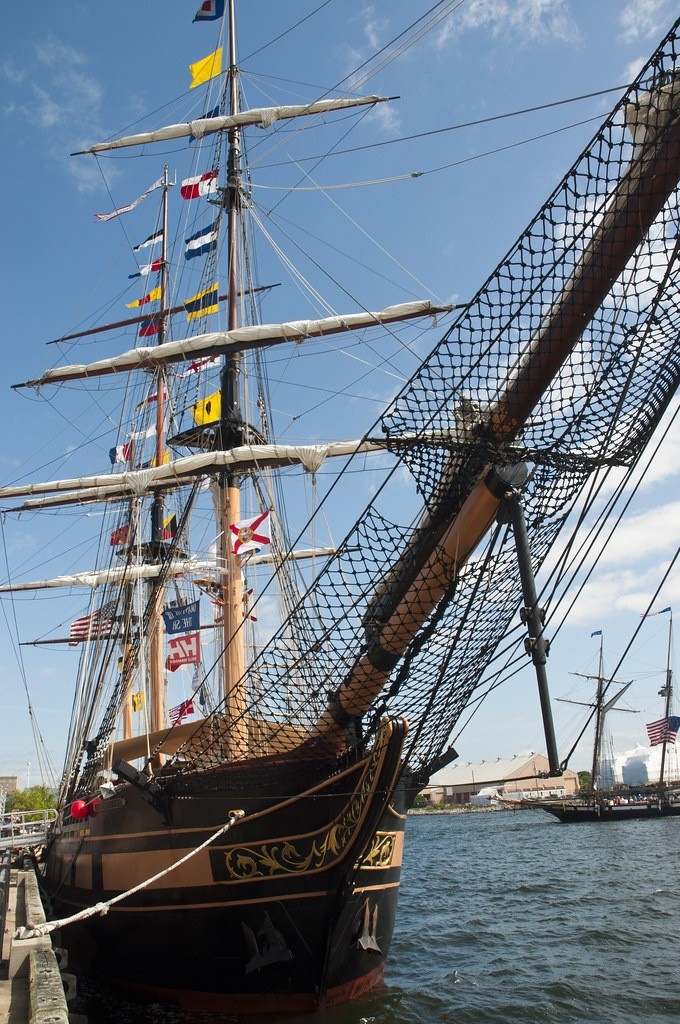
[0,1,678,1024]
[485,607,679,821]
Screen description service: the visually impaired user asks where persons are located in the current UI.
[614,793,680,804]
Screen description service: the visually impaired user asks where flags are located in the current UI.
[109,525,132,545]
[180,353,221,378]
[109,442,132,463]
[184,222,217,261]
[128,258,163,280]
[168,699,195,724]
[133,228,164,253]
[135,386,167,411]
[189,390,222,426]
[645,715,680,747]
[160,599,201,633]
[166,632,201,664]
[136,448,170,470]
[157,514,180,540]
[181,281,218,322]
[129,691,146,712]
[125,284,161,308]
[180,167,219,201]
[127,418,169,440]
[67,599,116,645]
[590,630,602,637]
[139,316,162,337]
[228,508,271,555]
[192,0,226,24]
[188,47,223,90]
